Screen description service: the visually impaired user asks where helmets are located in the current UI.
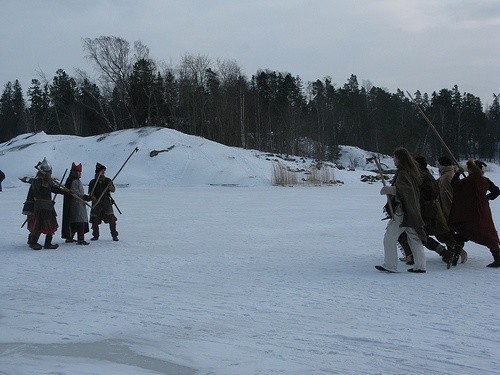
[40,156,52,174]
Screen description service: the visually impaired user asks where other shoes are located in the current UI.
[27,234,120,250]
[374,247,500,273]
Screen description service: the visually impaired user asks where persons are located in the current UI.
[374,147,427,273]
[61,161,95,245]
[398,156,455,270]
[452,158,500,267]
[18,157,74,250]
[88,161,122,241]
[436,154,464,267]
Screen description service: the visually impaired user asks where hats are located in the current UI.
[95,162,106,173]
[72,162,82,172]
[465,157,488,175]
